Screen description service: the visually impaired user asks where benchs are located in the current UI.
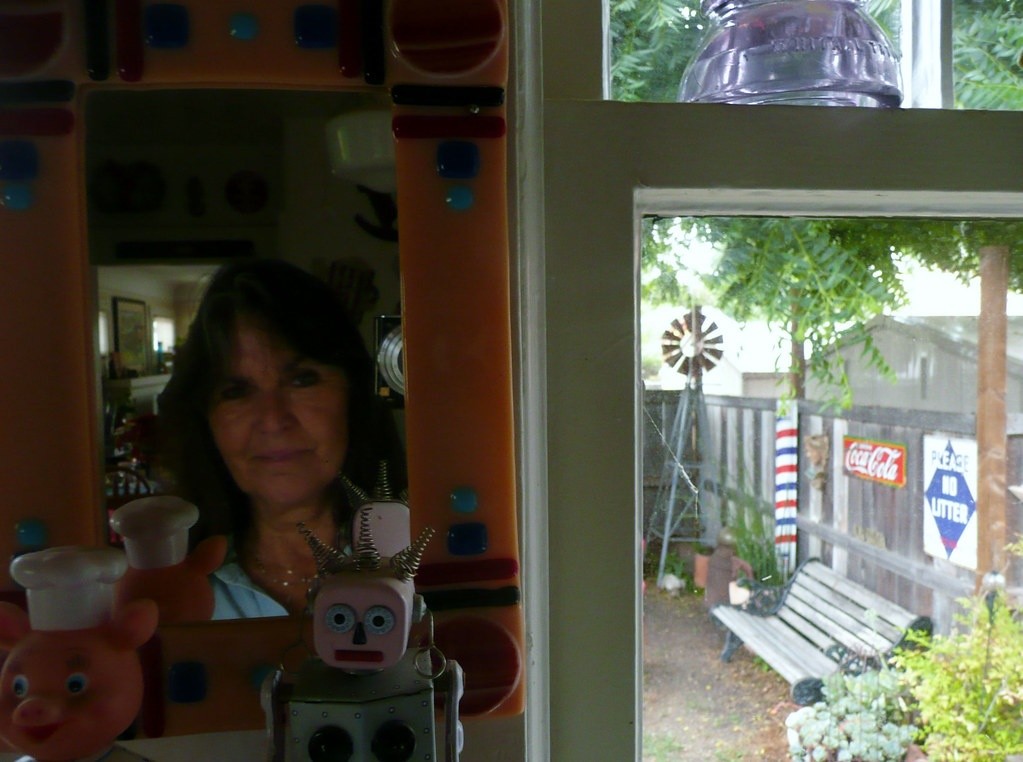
[707,557,935,709]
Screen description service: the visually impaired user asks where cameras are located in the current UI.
[375,315,406,407]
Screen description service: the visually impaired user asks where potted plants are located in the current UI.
[730,524,771,579]
[694,545,715,587]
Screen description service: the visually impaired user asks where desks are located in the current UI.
[100,372,171,415]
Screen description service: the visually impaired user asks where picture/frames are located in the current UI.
[113,296,148,371]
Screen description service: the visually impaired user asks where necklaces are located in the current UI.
[247,545,319,586]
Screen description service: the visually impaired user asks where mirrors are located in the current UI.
[0,0,527,741]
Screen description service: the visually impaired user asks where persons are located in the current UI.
[171,262,415,621]
